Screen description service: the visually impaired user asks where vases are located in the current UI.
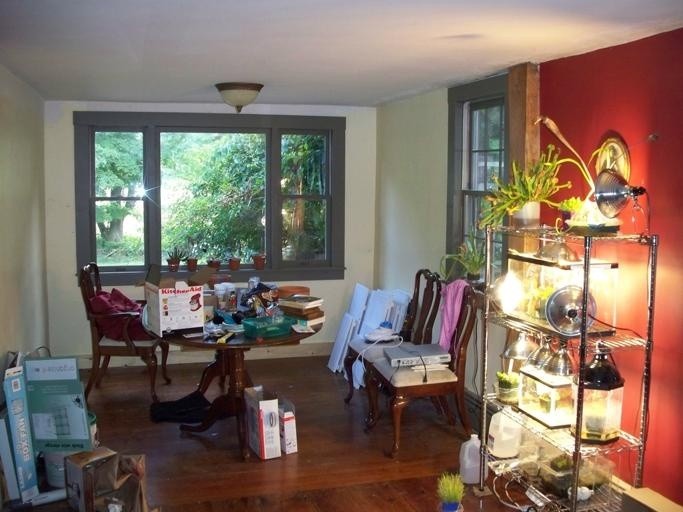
[185,254,265,271]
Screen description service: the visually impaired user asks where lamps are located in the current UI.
[499,332,576,379]
[544,283,644,338]
[212,78,264,117]
[532,238,585,264]
[592,165,653,234]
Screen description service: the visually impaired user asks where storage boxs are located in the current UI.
[141,276,208,338]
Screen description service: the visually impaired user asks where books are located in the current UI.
[278,294,325,327]
[410,363,450,373]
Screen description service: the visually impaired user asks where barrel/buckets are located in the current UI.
[487,405,521,457]
[460,434,488,485]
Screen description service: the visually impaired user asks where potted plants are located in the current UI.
[472,141,585,231]
[495,369,524,405]
[165,247,189,271]
[436,225,487,282]
[435,472,468,511]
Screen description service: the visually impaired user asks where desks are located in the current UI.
[140,303,323,463]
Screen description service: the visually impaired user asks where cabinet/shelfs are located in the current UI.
[476,216,660,511]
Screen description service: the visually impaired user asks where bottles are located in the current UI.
[214,282,237,313]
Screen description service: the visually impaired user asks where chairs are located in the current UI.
[75,264,170,404]
[342,267,480,459]
